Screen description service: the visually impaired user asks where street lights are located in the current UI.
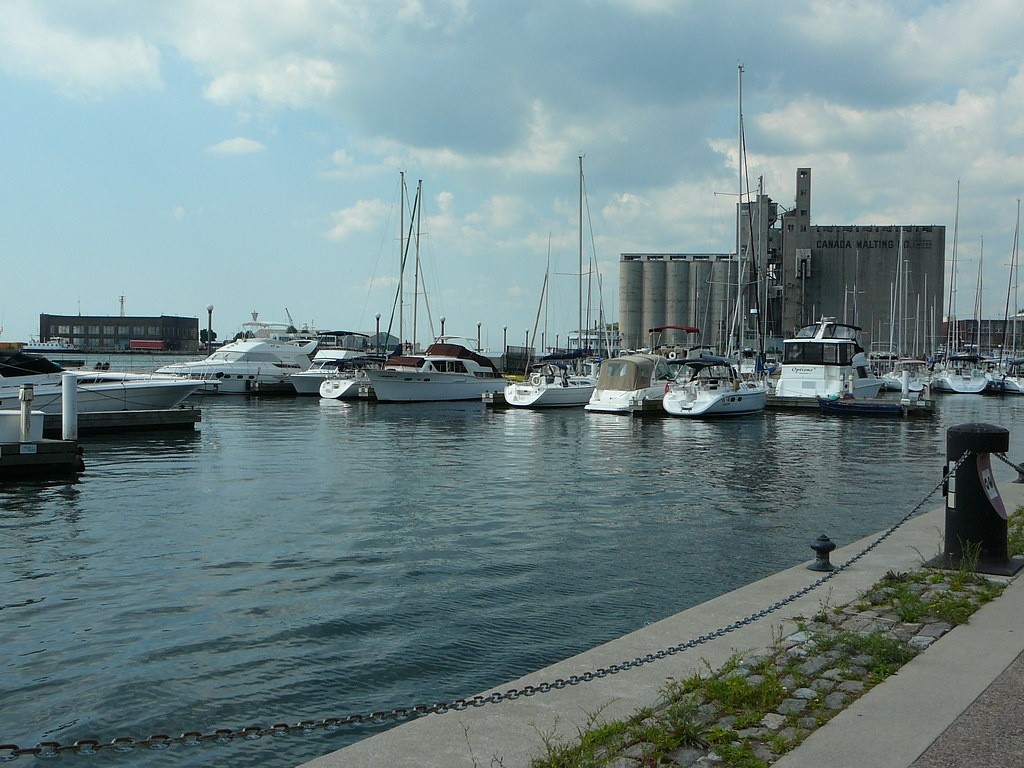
[524,328,529,355]
[477,322,483,352]
[503,325,507,352]
[556,332,559,353]
[567,334,570,353]
[207,304,214,357]
[374,311,381,357]
[541,331,545,353]
[438,316,446,344]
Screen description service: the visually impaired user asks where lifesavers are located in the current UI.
[531,375,541,386]
[668,351,676,359]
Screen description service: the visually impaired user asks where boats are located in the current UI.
[663,353,768,417]
[815,393,935,419]
[22,336,84,353]
[774,316,883,399]
[287,350,369,394]
[154,321,318,395]
[0,409,86,483]
[0,348,224,411]
[584,353,676,412]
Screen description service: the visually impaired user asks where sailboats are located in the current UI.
[318,171,511,402]
[503,229,596,408]
[868,179,1024,393]
